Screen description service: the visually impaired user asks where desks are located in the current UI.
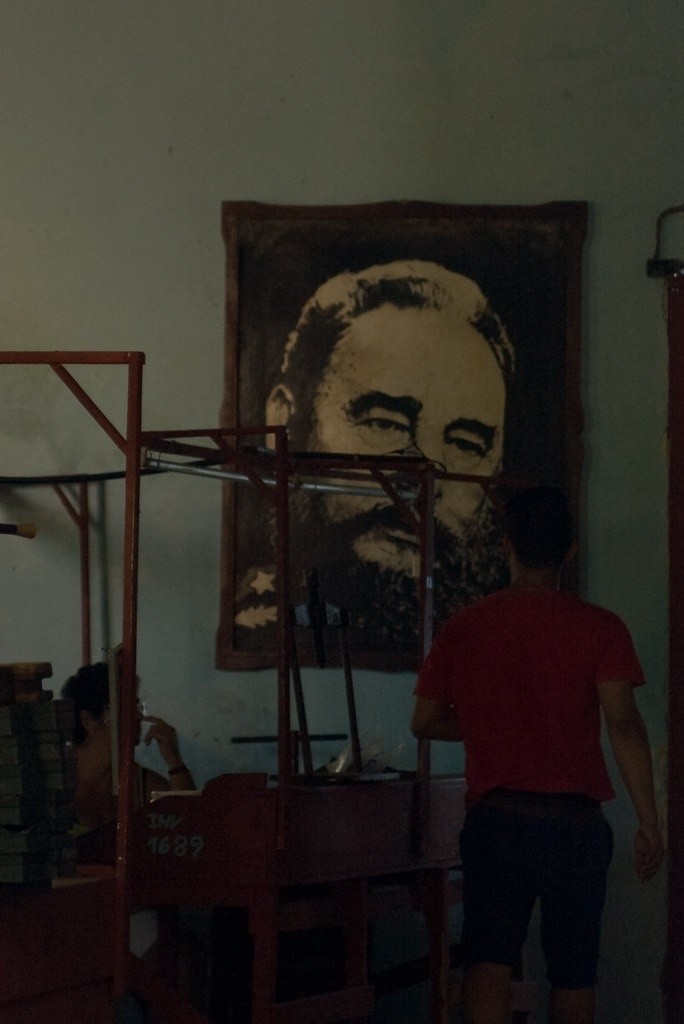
[0,866,116,1024]
[77,770,540,1024]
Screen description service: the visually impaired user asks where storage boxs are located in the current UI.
[0,698,79,886]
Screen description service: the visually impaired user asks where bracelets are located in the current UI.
[167,764,187,776]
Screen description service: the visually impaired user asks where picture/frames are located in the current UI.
[213,200,589,674]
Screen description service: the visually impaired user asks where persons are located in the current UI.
[410,487,667,1024]
[45,661,258,1023]
